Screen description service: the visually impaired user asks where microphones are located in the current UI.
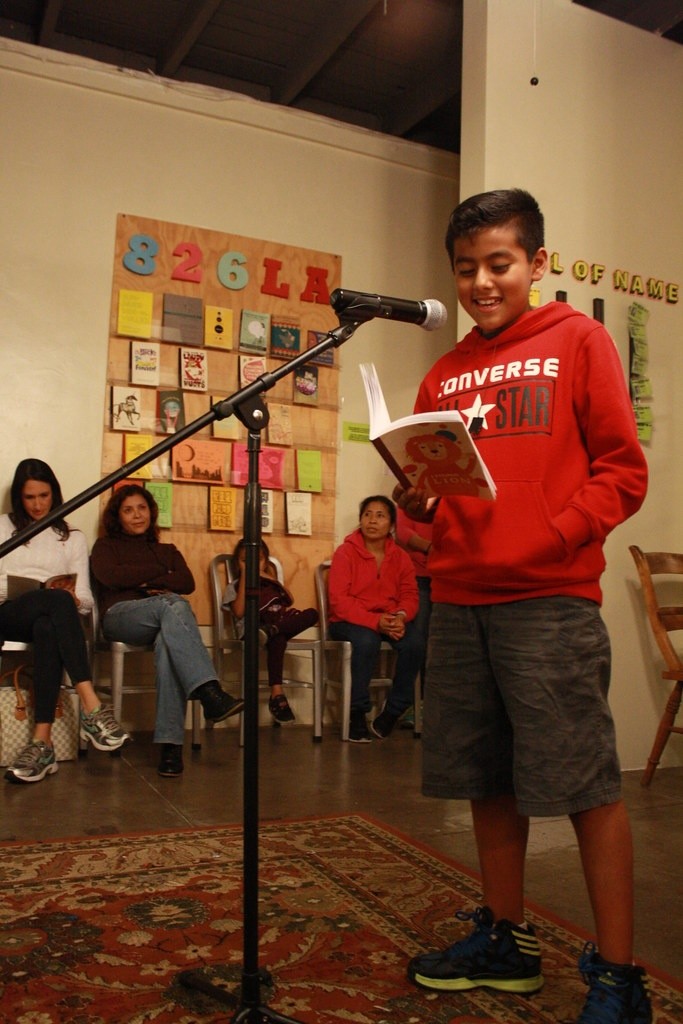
[330,288,448,330]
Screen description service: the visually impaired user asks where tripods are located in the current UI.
[1,292,381,1024]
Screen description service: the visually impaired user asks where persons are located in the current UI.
[0,458,134,781]
[392,189,652,1024]
[89,484,243,777]
[221,538,320,723]
[327,495,433,741]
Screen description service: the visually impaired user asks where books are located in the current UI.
[110,289,334,536]
[7,574,76,598]
[360,363,497,500]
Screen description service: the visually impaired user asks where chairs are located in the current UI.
[629,545,683,785]
[2,553,421,755]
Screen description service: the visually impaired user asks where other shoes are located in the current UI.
[269,694,297,725]
[258,629,268,649]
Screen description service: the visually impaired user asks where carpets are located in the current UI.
[0,813,683,1024]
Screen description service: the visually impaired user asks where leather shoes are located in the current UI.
[200,685,244,723]
[158,742,183,778]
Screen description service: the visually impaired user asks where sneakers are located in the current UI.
[407,919,543,990]
[349,711,371,743]
[81,704,133,751]
[5,740,58,781]
[372,694,398,738]
[575,952,652,1024]
